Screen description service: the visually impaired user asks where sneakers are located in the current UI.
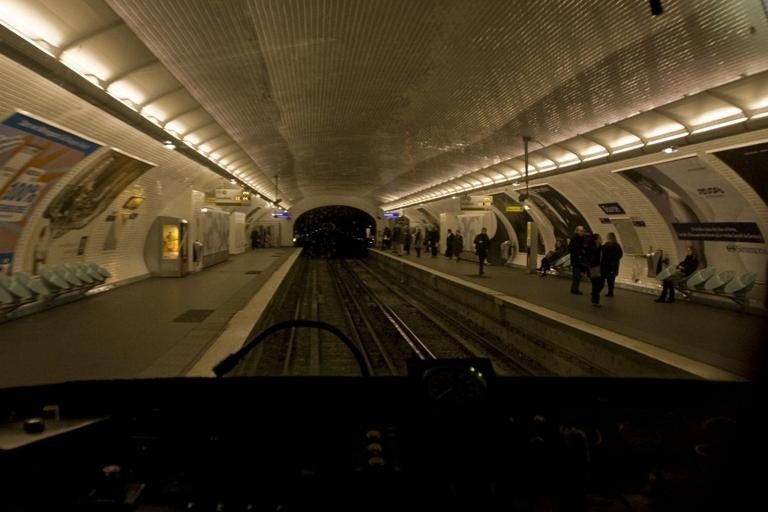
[665,299,676,304]
[536,269,543,271]
[540,274,547,277]
[570,287,615,308]
[654,298,666,303]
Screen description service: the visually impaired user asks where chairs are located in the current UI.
[1,258,111,315]
[655,264,759,310]
[552,252,572,278]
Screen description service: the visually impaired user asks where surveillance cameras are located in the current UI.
[519,193,529,201]
[274,199,281,205]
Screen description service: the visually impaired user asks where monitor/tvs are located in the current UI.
[598,203,625,214]
[124,196,145,209]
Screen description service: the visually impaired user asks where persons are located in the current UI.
[653,246,700,303]
[250,225,273,250]
[382,224,624,308]
